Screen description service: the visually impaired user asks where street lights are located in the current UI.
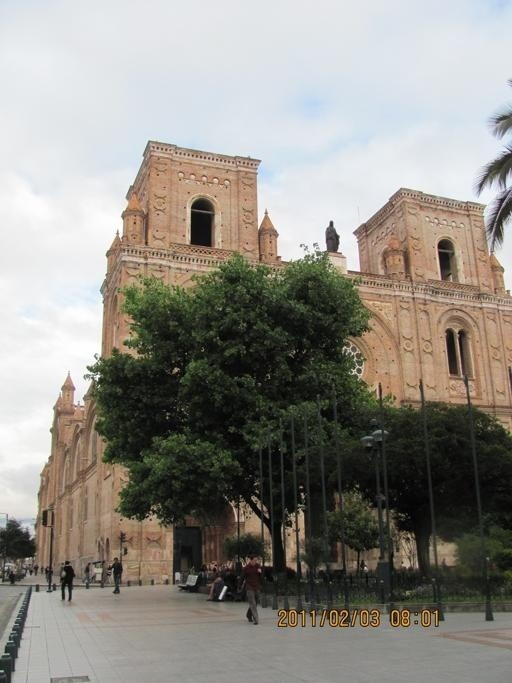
[359,417,397,602]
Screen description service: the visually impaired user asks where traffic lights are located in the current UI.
[121,531,126,542]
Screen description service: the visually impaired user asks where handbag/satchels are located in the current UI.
[258,573,266,592]
[61,570,66,578]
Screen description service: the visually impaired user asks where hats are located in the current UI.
[65,561,71,565]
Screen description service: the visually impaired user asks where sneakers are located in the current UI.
[247,616,258,623]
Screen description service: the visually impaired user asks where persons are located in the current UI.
[5,561,112,584]
[373,556,448,601]
[191,562,246,602]
[325,220,340,252]
[108,557,123,594]
[60,561,75,602]
[359,559,367,571]
[241,555,264,625]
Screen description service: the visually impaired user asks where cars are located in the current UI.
[5,562,13,571]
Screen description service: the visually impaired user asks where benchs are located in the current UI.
[178,574,202,593]
